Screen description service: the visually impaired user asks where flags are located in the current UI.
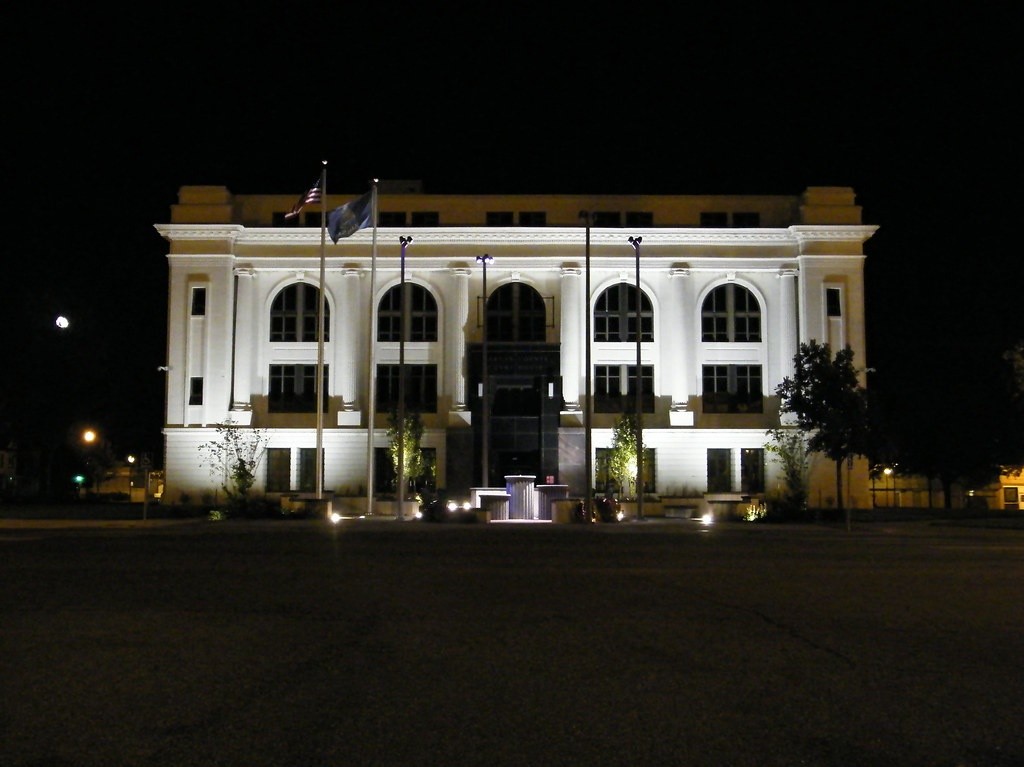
[326,191,373,244]
[284,176,322,218]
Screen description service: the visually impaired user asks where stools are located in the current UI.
[470,508,492,522]
[549,498,580,522]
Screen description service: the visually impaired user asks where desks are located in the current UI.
[479,494,512,520]
[536,484,568,520]
[504,475,536,520]
[468,487,506,509]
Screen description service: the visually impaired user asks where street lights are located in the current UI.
[628,235,645,521]
[396,235,414,522]
[475,253,495,488]
[127,454,136,504]
[884,468,892,508]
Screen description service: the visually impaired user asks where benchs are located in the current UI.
[280,494,333,518]
